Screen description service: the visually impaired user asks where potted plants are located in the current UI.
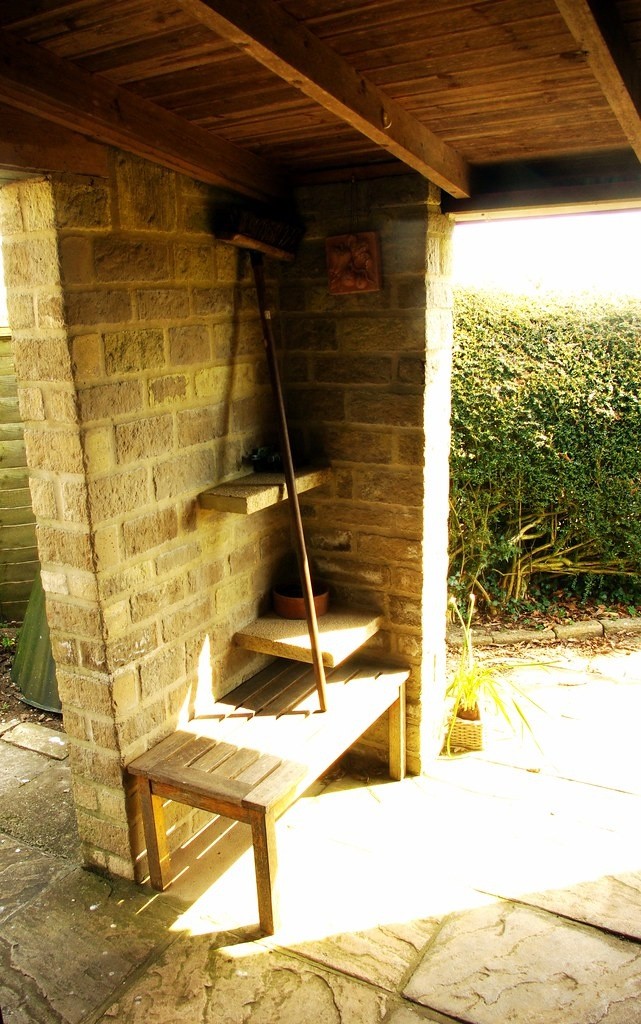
[440,594,568,758]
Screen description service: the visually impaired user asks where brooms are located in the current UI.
[196,204,333,719]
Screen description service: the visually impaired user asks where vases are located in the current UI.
[271,581,329,620]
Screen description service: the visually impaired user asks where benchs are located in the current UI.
[127,657,411,935]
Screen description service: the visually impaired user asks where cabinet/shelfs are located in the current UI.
[197,461,382,667]
